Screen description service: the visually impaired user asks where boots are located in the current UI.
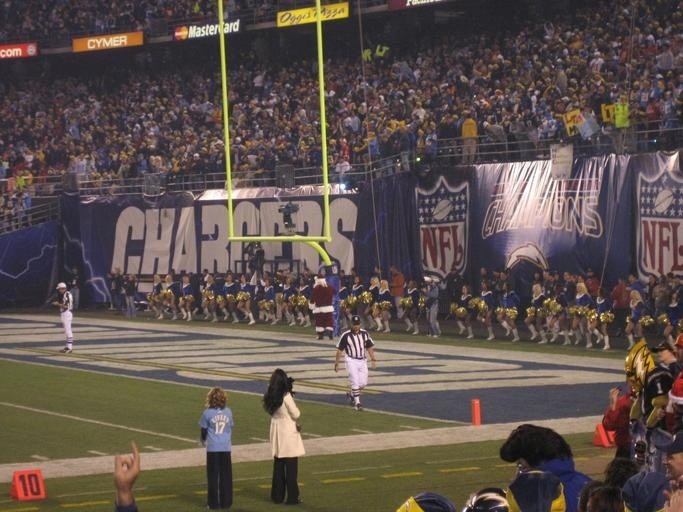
[151,305,311,328]
[627,333,635,351]
[456,320,611,351]
[339,313,420,335]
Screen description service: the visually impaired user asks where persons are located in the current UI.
[198,386,233,512]
[1,1,681,353]
[112,440,142,512]
[395,491,457,512]
[462,353,683,512]
[261,368,308,506]
[332,315,377,412]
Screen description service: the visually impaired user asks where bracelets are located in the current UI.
[370,359,376,362]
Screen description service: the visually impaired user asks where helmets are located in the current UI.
[675,333,683,348]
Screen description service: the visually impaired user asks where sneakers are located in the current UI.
[61,347,72,354]
[347,393,363,411]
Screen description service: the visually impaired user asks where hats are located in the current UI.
[649,428,682,454]
[396,468,672,512]
[351,315,362,324]
[651,341,673,352]
[314,274,326,281]
[55,282,66,290]
